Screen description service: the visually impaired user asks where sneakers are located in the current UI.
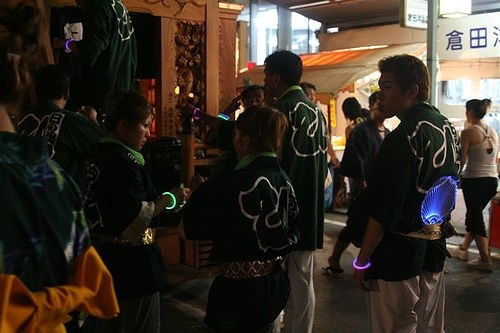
[466,258,493,271]
[446,244,468,261]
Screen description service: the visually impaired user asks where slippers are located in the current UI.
[321,264,348,278]
